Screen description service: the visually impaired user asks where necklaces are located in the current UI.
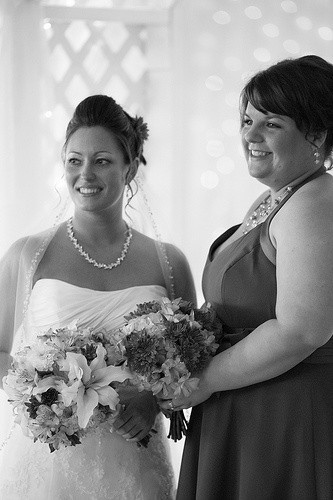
[241,185,299,237]
[67,217,132,270]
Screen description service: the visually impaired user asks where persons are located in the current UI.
[155,55,333,500]
[0,95,197,500]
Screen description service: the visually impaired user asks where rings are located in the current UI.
[168,400,175,409]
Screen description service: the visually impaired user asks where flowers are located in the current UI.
[119,297,222,442]
[2,326,127,453]
